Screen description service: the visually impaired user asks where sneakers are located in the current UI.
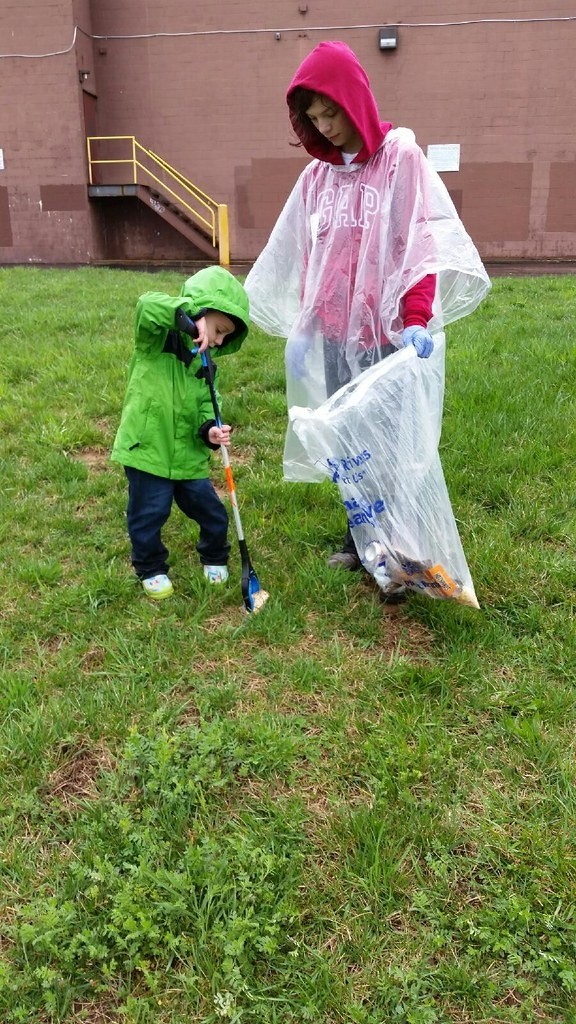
[141,574,175,600]
[204,565,229,585]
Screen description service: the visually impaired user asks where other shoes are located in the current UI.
[380,590,405,602]
[327,551,358,572]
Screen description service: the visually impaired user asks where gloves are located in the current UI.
[403,325,434,358]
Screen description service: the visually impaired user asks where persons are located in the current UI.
[284,39,439,605]
[110,266,250,601]
